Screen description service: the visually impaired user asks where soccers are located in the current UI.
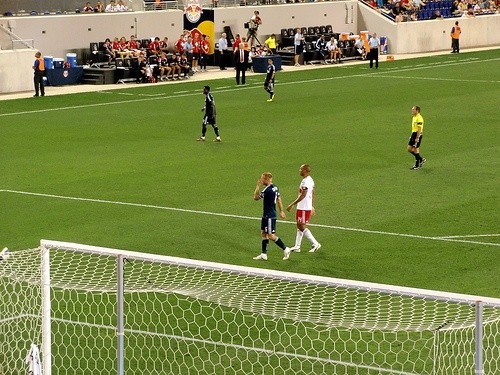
[0,246,10,260]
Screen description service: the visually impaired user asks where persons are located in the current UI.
[450,20,461,54]
[294,29,305,67]
[286,164,321,252]
[212,0,329,8]
[364,0,443,22]
[219,32,227,70]
[197,86,221,142]
[408,105,426,170]
[264,58,275,101]
[451,0,500,17]
[233,11,270,71]
[83,0,128,12]
[235,43,248,84]
[368,32,380,69]
[31,51,45,96]
[153,0,163,10]
[103,33,210,83]
[253,172,293,260]
[265,34,277,54]
[316,34,367,64]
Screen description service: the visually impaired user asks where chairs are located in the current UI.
[281,25,358,61]
[416,0,454,20]
[90,40,190,83]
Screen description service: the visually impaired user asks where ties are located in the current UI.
[241,49,243,61]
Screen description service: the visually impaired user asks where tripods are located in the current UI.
[246,30,261,47]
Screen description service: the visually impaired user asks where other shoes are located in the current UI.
[295,63,300,67]
[205,69,208,72]
[165,77,169,81]
[160,77,163,81]
[173,78,176,80]
[201,70,203,72]
[195,68,199,72]
[185,75,190,79]
[178,77,183,80]
[193,69,195,72]
[321,58,343,65]
[220,68,227,70]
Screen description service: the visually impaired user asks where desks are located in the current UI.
[252,54,282,73]
[43,66,83,86]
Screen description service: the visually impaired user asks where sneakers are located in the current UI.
[410,165,419,170]
[308,242,321,253]
[267,99,272,101]
[212,138,220,142]
[291,246,300,253]
[253,255,267,260]
[197,136,205,141]
[272,94,275,100]
[283,249,293,260]
[420,158,426,168]
